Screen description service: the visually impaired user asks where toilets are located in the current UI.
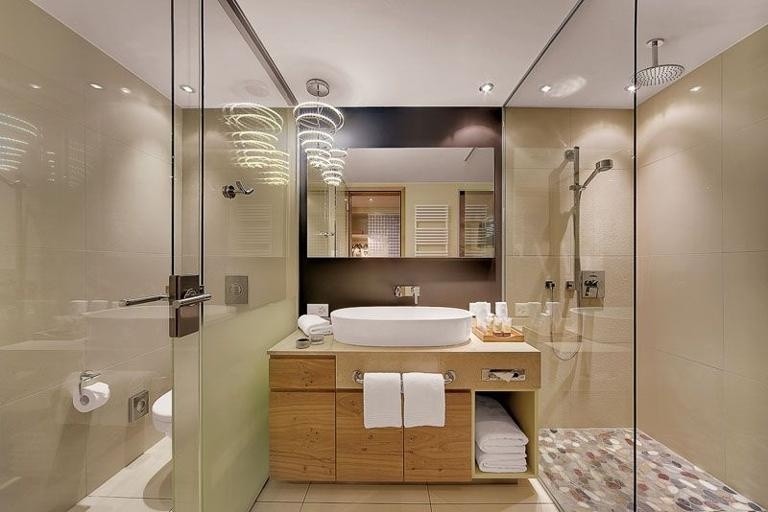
[151,388,174,439]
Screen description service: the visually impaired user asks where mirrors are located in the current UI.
[306,147,496,258]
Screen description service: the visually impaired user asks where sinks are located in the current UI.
[568,304,635,345]
[331,306,472,348]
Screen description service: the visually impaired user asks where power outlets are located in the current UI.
[514,302,529,319]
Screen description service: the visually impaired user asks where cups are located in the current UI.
[486,316,512,333]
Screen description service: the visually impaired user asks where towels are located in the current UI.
[296,314,333,337]
[361,369,448,431]
[474,394,530,476]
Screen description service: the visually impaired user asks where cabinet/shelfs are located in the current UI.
[264,327,541,485]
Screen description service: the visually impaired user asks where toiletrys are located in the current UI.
[295,337,310,348]
[309,333,325,346]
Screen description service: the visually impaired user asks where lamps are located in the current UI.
[319,148,348,185]
[292,79,345,167]
[222,80,289,186]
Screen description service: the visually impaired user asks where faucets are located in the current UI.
[395,285,421,305]
[581,268,606,301]
